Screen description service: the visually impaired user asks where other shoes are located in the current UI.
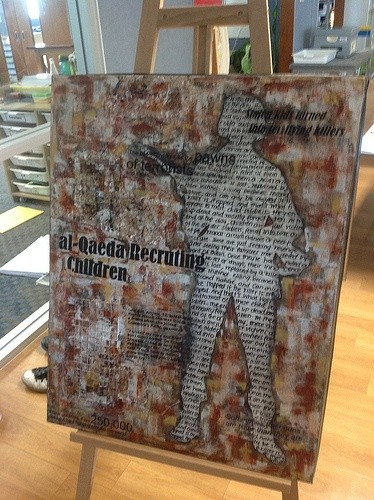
[22,366,47,392]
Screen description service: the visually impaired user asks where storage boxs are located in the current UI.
[311,26,358,58]
[292,47,340,65]
[0,109,50,197]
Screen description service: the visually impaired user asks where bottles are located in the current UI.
[49,58,58,84]
[59,56,70,75]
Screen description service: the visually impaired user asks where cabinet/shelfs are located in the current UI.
[0,0,80,202]
